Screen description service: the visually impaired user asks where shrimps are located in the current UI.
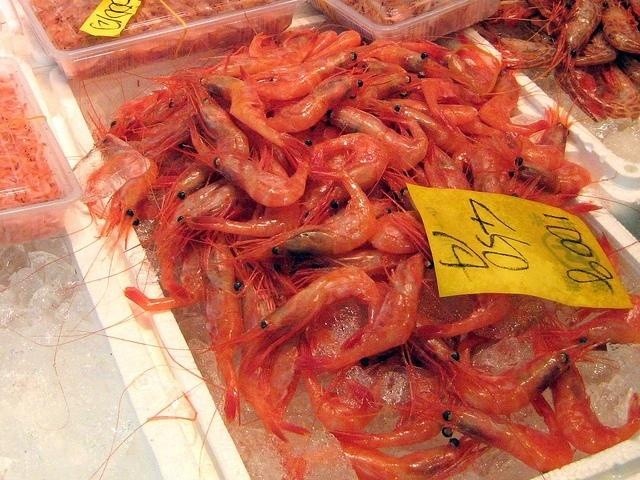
[0,0,640,479]
